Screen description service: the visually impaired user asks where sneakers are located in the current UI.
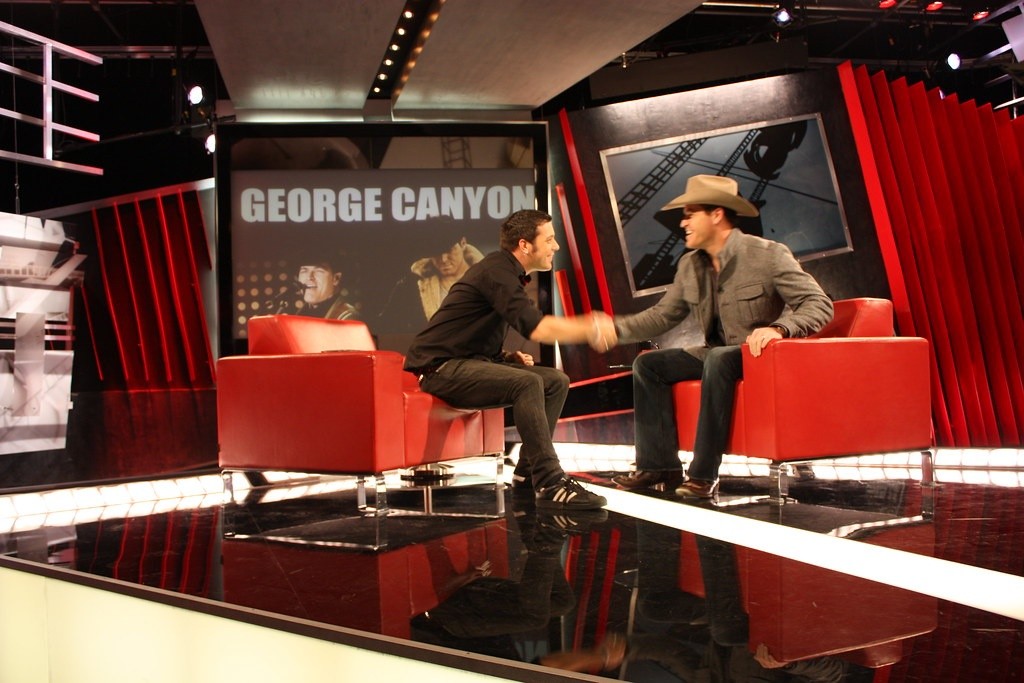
[511,474,533,493]
[510,495,536,524]
[533,507,608,547]
[534,472,608,509]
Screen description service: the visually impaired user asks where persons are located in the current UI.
[382,216,485,329]
[408,488,875,682]
[588,173,834,499]
[403,209,606,510]
[291,250,361,322]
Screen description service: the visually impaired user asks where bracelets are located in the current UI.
[600,646,610,669]
[592,319,601,343]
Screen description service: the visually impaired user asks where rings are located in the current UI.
[761,335,766,339]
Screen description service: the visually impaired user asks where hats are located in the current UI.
[660,174,759,217]
[292,244,343,273]
[419,214,462,259]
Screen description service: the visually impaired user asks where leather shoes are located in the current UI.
[612,467,683,490]
[674,475,720,499]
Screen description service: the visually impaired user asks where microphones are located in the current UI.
[716,286,722,293]
[267,280,304,307]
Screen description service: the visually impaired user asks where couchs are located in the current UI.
[670,298,935,546]
[216,314,505,551]
